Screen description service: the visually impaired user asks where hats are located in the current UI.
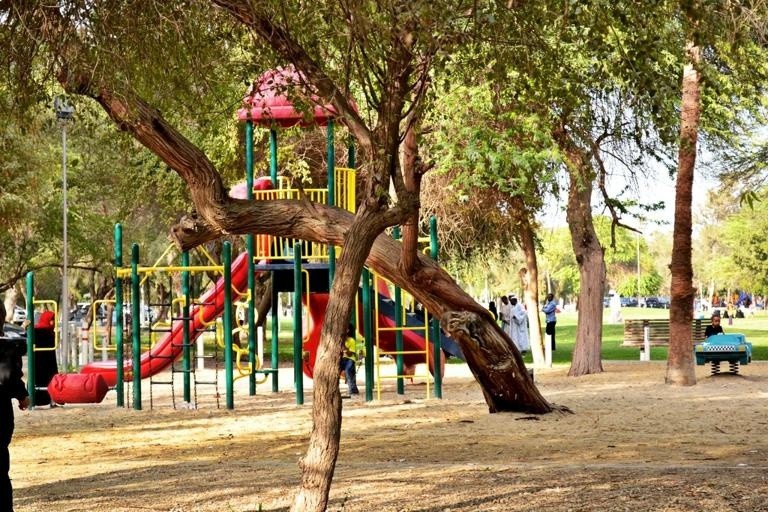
[508,294,517,300]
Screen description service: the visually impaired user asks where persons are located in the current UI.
[0,299,30,512]
[489,294,557,355]
[342,323,359,395]
[33,310,59,409]
[704,293,752,338]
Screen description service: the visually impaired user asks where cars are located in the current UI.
[601,290,670,309]
[10,301,157,329]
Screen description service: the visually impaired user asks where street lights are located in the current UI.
[52,89,76,376]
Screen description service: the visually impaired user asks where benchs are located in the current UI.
[618,317,715,364]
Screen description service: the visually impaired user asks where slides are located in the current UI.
[82,251,257,386]
